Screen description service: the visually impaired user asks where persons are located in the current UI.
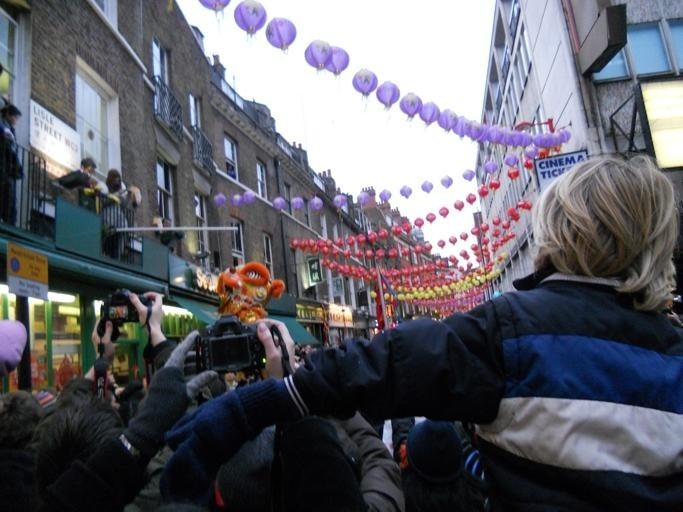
[163,155,681,512]
[91,169,128,206]
[0,291,488,511]
[56,156,96,194]
[0,101,25,226]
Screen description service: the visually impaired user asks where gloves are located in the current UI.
[119,330,219,465]
[158,376,290,504]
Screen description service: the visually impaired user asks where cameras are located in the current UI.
[200,314,275,374]
[104,288,152,324]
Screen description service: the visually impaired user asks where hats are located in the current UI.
[32,389,57,414]
[213,430,276,511]
[406,419,464,483]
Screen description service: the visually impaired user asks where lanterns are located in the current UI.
[201,1,230,22]
[214,194,227,206]
[305,41,349,76]
[291,175,574,324]
[377,81,399,110]
[266,18,296,51]
[399,92,470,138]
[272,197,286,210]
[232,191,256,207]
[463,120,571,180]
[234,2,267,36]
[353,69,379,98]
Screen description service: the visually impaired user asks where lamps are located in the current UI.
[514,117,555,134]
[192,250,209,266]
[303,285,315,297]
